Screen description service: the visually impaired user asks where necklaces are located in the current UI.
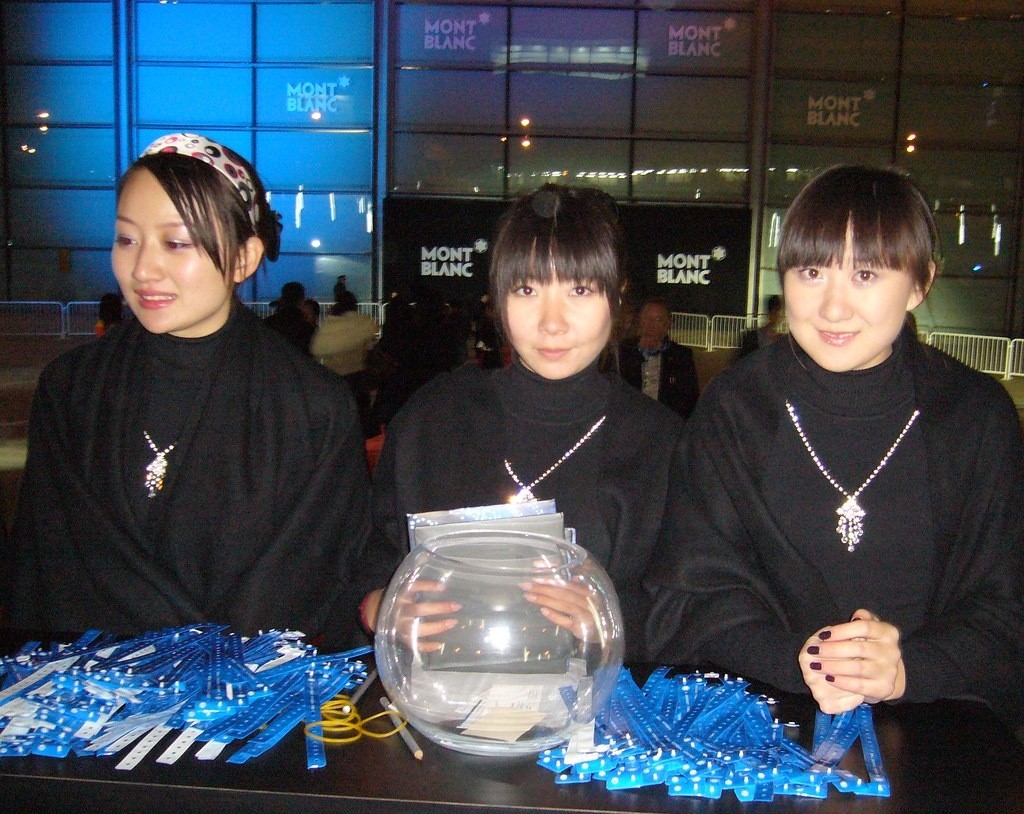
[504,416,607,503]
[785,398,920,552]
[141,429,179,499]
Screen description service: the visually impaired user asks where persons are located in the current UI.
[0,131,370,654]
[636,161,1024,716]
[94,275,788,423]
[338,181,687,669]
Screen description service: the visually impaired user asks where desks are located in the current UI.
[0,666,1024,814]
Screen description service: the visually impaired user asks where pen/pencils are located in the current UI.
[342,666,379,715]
[379,695,424,762]
